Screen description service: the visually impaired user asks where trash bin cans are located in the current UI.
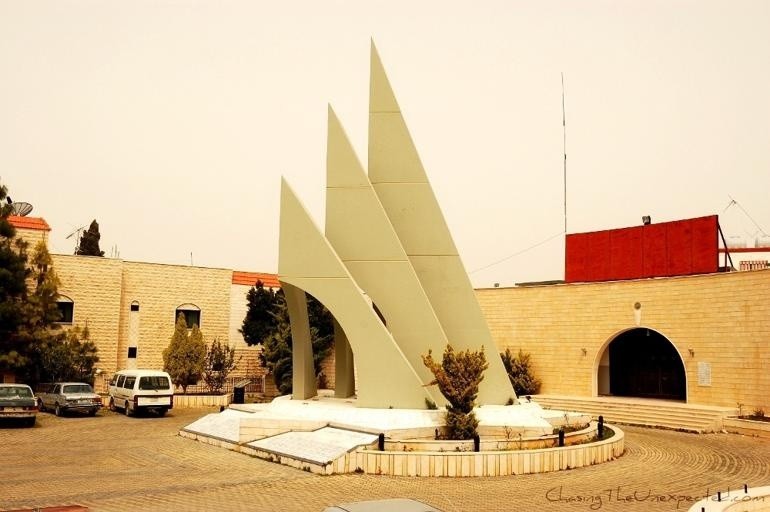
[234,387,244,403]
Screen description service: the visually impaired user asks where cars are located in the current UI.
[39,381,103,416]
[0,383,38,424]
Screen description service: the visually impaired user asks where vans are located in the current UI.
[107,371,174,415]
